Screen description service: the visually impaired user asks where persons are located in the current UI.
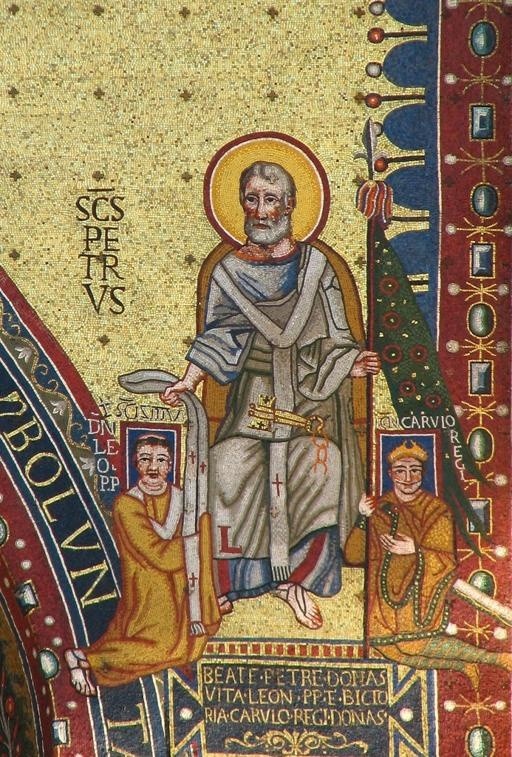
[342,441,512,688]
[162,161,382,631]
[63,434,221,697]
